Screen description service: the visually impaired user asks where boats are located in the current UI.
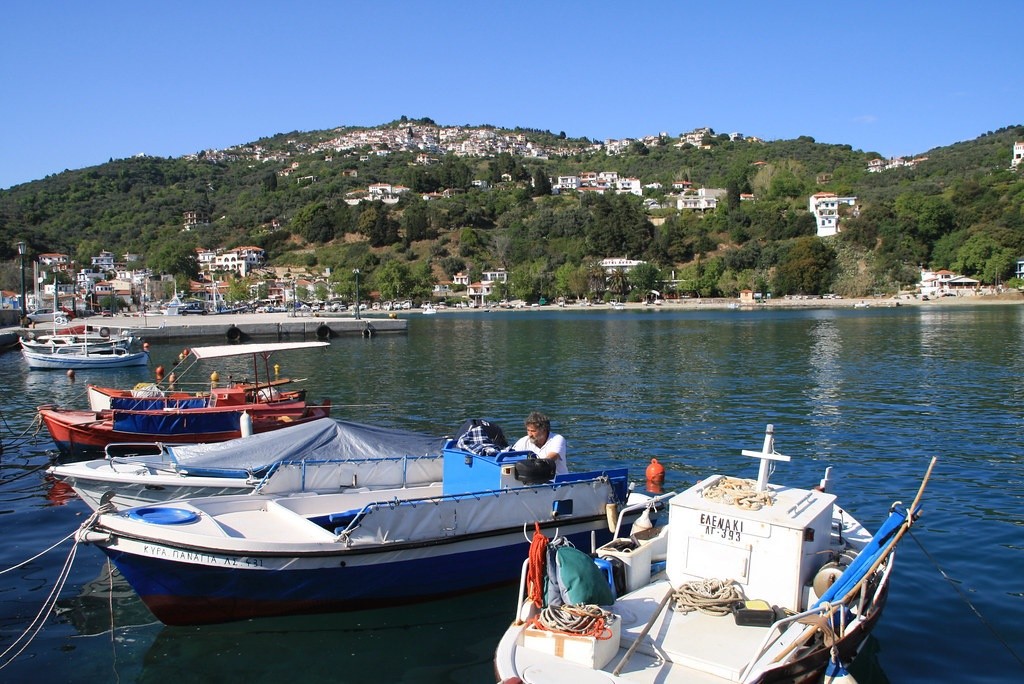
[423,309,437,315]
[36,342,331,454]
[20,318,150,370]
[493,425,937,684]
[75,438,664,626]
[19,296,134,354]
[45,417,508,511]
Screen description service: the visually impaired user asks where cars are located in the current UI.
[102,310,112,317]
[27,308,69,324]
[59,306,75,321]
[179,306,208,316]
[256,299,539,314]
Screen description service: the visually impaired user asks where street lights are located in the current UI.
[353,267,360,319]
[17,242,29,328]
[51,263,61,308]
[293,279,295,316]
[71,273,78,312]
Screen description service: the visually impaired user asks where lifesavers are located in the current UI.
[99,327,110,337]
[55,317,63,325]
[60,318,68,325]
[362,329,372,337]
[225,326,242,343]
[315,324,331,340]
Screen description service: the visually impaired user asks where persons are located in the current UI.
[512,412,569,483]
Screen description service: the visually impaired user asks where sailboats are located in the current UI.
[203,275,248,315]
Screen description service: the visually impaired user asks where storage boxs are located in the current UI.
[524,614,621,670]
[596,538,651,592]
